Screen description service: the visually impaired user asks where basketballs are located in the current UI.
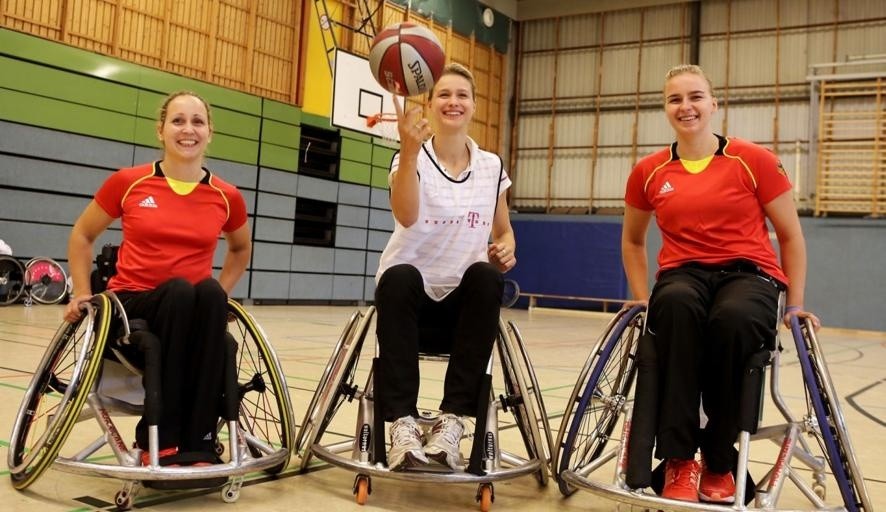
[369,21,444,96]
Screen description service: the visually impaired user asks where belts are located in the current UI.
[680,258,780,284]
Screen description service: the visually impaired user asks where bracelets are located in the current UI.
[784,304,804,313]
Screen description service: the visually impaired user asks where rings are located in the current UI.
[502,250,506,257]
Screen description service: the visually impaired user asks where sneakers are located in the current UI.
[180,439,221,470]
[423,412,465,471]
[133,433,183,469]
[698,450,736,505]
[659,454,700,504]
[386,413,429,472]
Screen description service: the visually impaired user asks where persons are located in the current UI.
[619,64,821,507]
[60,91,254,489]
[372,60,516,473]
